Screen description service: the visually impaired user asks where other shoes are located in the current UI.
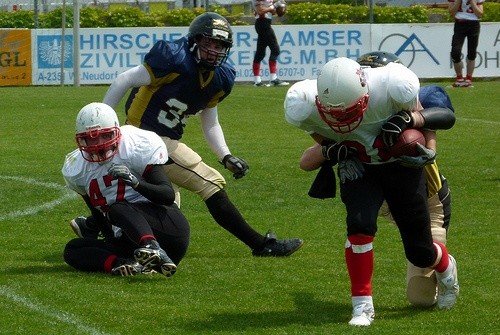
[450,81,472,88]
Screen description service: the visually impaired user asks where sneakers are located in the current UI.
[112,262,157,276]
[269,78,289,86]
[70,216,99,240]
[134,241,176,277]
[254,82,270,88]
[434,254,460,309]
[348,304,373,326]
[251,233,303,257]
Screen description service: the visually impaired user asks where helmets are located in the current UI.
[75,102,119,165]
[317,57,369,133]
[357,52,403,69]
[188,12,234,71]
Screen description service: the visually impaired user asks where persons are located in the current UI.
[298,51,457,307]
[69,11,304,258]
[284,50,459,326]
[253,0,290,87]
[63,101,190,277]
[447,0,484,88]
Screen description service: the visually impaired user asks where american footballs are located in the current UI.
[396,130,425,156]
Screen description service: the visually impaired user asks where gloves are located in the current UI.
[396,142,437,170]
[381,108,414,147]
[339,156,363,185]
[218,154,248,180]
[321,142,356,161]
[107,162,138,188]
